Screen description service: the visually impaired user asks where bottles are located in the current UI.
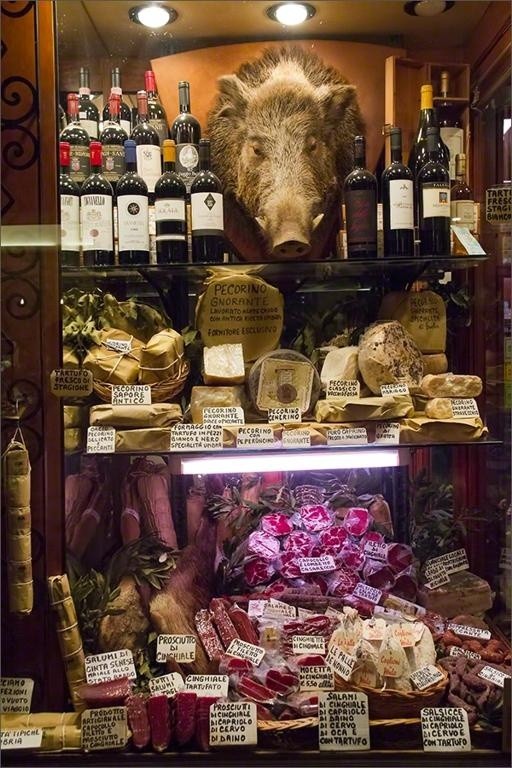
[154,139,189,270]
[190,138,223,269]
[381,126,415,258]
[417,126,452,257]
[116,139,150,271]
[433,70,465,189]
[407,84,450,177]
[450,153,475,257]
[135,70,169,161]
[58,93,91,205]
[59,141,80,271]
[344,135,379,258]
[80,141,115,271]
[129,89,162,204]
[102,67,131,141]
[170,80,201,205]
[76,65,99,142]
[98,93,129,206]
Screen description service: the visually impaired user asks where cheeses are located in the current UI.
[422,374,483,398]
[191,386,246,424]
[248,349,320,417]
[320,347,359,385]
[358,322,425,395]
[197,273,283,363]
[202,343,245,385]
[426,399,453,419]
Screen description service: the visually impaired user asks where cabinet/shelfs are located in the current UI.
[40,0,512,764]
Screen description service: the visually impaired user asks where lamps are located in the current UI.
[128,2,179,29]
[266,1,317,26]
[404,0,455,18]
